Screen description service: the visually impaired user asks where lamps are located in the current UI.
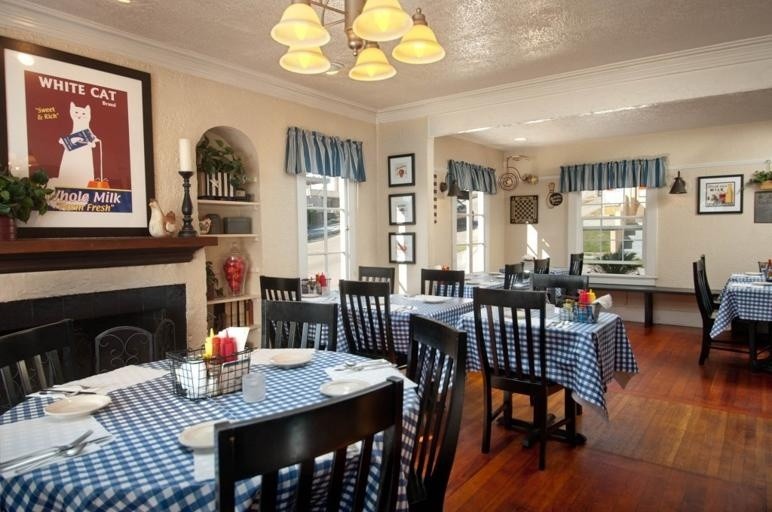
[269,0,447,84]
[666,169,688,195]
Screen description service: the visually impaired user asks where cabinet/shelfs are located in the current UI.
[196,200,260,350]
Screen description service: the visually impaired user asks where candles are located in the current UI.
[180,136,193,171]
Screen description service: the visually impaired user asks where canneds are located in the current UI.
[60,128,95,152]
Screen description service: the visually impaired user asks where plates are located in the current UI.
[301,293,319,297]
[465,271,505,284]
[415,296,451,302]
[504,310,526,319]
[178,420,233,449]
[371,298,385,305]
[44,395,112,415]
[752,282,772,286]
[270,349,312,366]
[745,272,763,275]
[320,379,369,397]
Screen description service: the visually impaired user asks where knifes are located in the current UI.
[0,435,111,472]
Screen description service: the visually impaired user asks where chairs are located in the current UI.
[692,254,759,371]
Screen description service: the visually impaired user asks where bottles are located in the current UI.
[223,242,248,297]
[316,272,327,286]
[205,328,237,363]
[719,188,726,204]
[726,184,733,203]
[578,288,596,305]
[766,259,772,281]
[560,307,570,321]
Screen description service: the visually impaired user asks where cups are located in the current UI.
[322,287,330,297]
[242,373,266,403]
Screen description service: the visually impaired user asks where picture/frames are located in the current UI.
[387,191,417,226]
[389,231,417,264]
[387,153,415,186]
[0,34,155,236]
[697,174,744,214]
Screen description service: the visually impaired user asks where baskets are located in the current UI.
[560,302,600,323]
[166,346,253,400]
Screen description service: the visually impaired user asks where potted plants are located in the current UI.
[0,163,54,243]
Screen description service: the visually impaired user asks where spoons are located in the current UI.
[15,442,87,473]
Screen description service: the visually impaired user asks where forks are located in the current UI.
[0,430,94,469]
